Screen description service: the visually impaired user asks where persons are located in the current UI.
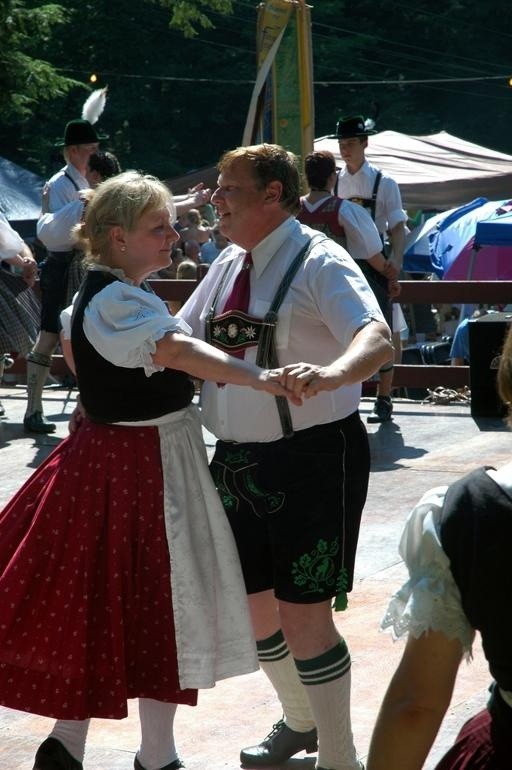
[0,113,510,435]
[0,168,312,762]
[361,324,510,764]
[164,141,392,762]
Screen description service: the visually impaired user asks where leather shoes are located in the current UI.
[33,738,83,770]
[368,396,393,423]
[24,412,56,433]
[240,719,318,763]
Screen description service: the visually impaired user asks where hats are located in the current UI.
[326,115,378,139]
[54,120,110,147]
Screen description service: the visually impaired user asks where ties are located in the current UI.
[216,253,253,388]
